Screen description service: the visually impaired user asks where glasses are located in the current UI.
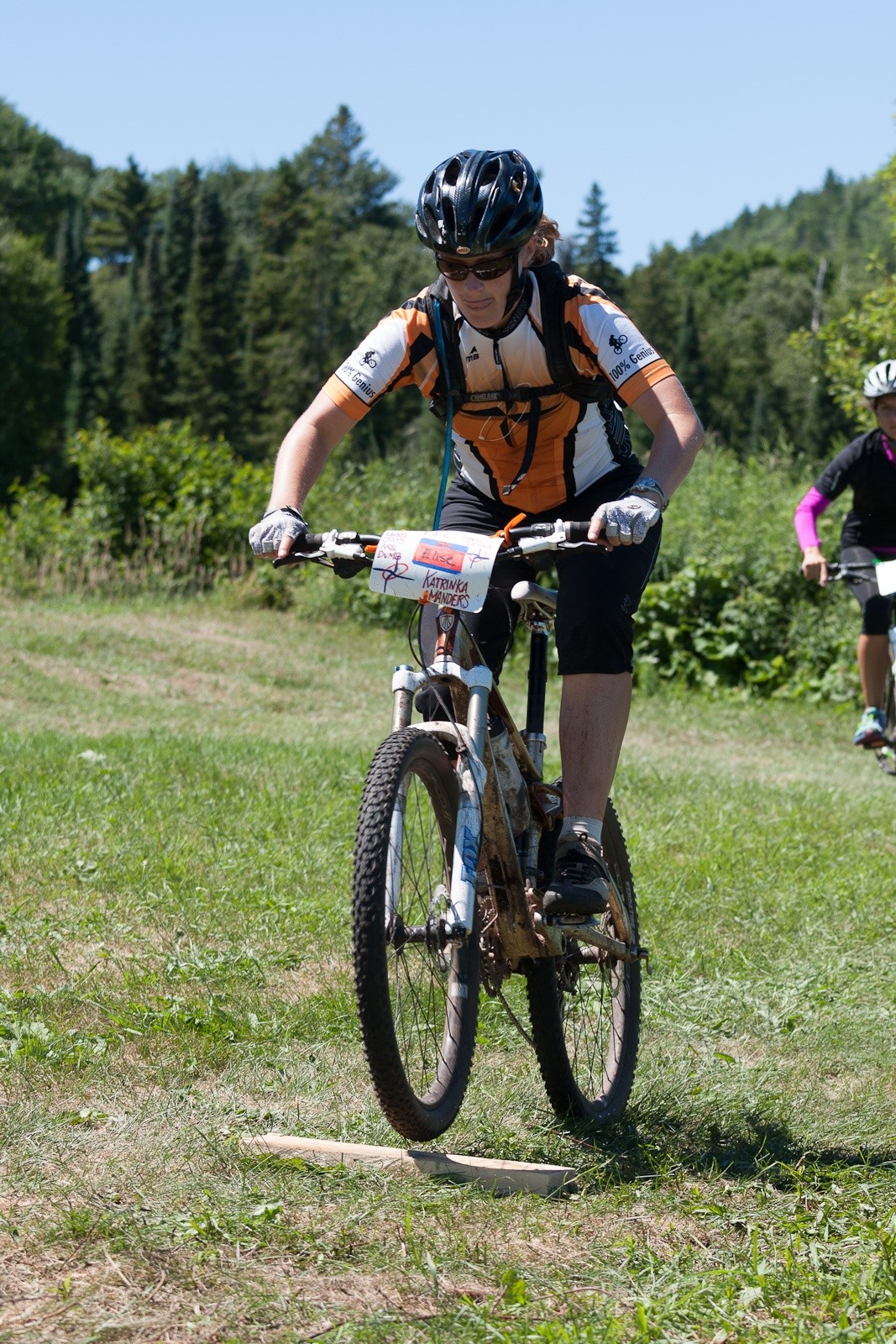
[430,252,518,281]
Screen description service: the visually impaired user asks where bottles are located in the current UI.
[485,716,531,837]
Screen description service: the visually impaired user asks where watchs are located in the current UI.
[629,477,665,503]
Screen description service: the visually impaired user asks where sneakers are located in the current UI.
[851,706,891,747]
[543,834,615,918]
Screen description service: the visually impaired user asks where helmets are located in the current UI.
[411,147,545,258]
[861,358,896,396]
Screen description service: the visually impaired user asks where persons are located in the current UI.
[248,148,703,969]
[794,360,896,746]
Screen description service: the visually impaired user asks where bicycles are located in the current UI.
[799,559,896,778]
[272,516,652,1145]
